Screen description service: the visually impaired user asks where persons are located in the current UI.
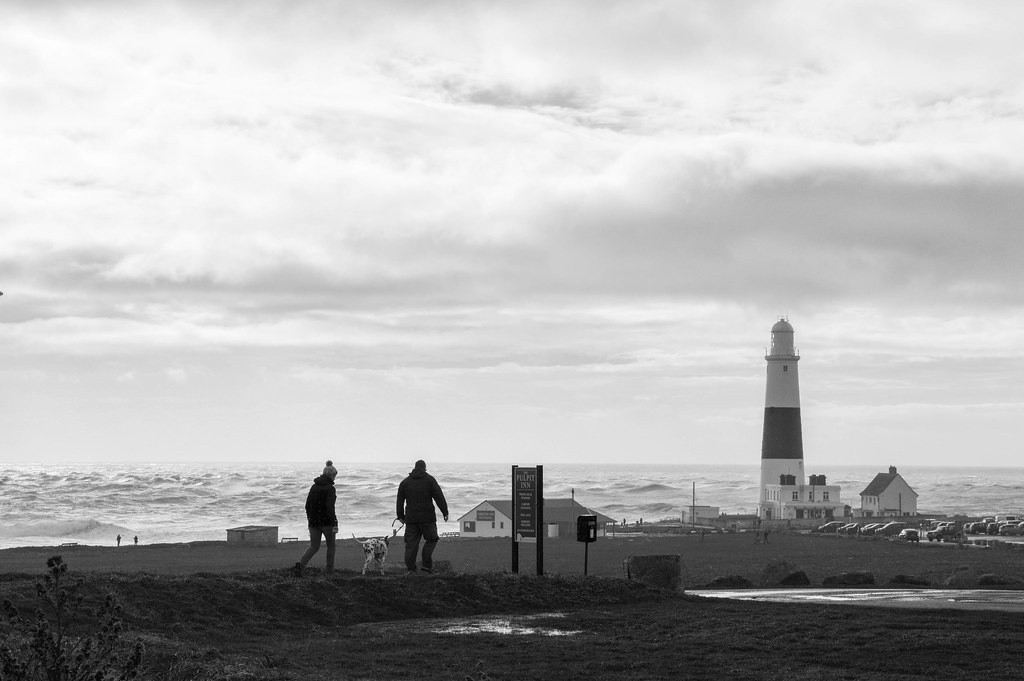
[295,461,341,578]
[764,530,769,544]
[134,536,138,546]
[117,535,121,546]
[396,460,449,574]
[753,532,760,544]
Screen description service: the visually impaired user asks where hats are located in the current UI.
[323,461,338,476]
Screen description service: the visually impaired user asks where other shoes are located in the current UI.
[407,566,434,577]
[294,561,304,578]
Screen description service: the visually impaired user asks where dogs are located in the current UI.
[351,532,390,578]
